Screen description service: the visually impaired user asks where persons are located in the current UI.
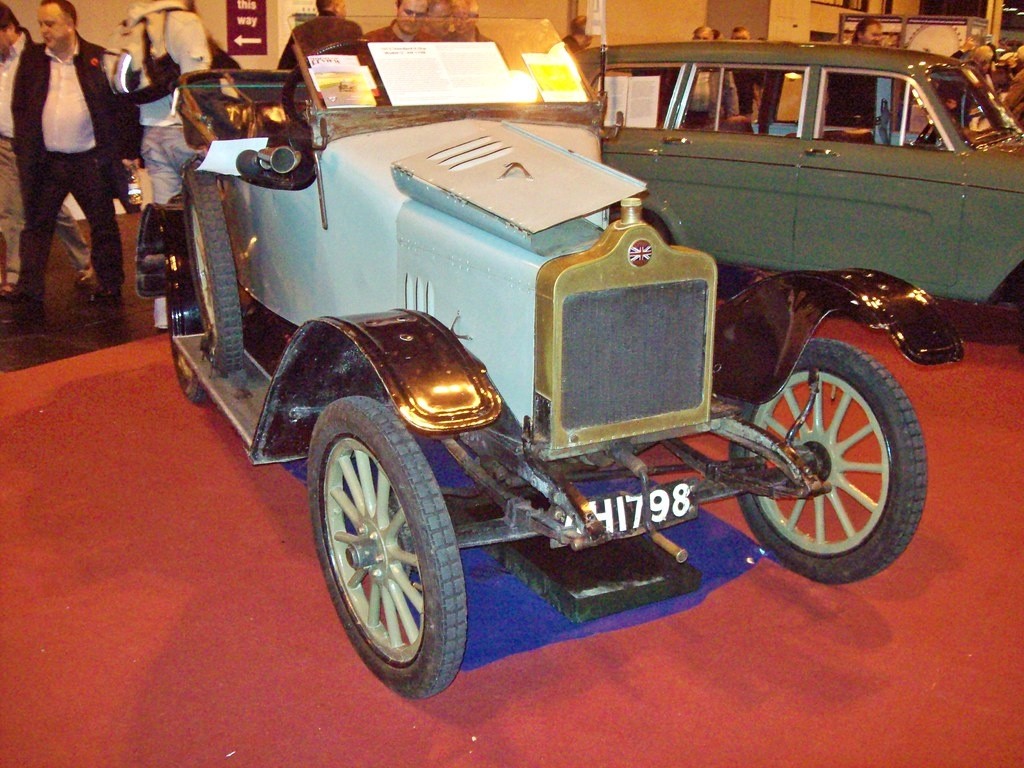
[356,0,444,68]
[278,0,365,69]
[425,0,454,42]
[103,0,241,333]
[825,17,883,145]
[933,37,1024,130]
[0,0,141,306]
[681,26,768,132]
[443,0,506,57]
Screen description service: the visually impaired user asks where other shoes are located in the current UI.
[74,279,95,290]
[0,305,46,324]
[0,283,18,298]
[90,288,122,302]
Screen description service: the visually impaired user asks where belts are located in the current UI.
[41,147,97,160]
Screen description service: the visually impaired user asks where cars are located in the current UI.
[574,38,1024,310]
[136,12,967,702]
